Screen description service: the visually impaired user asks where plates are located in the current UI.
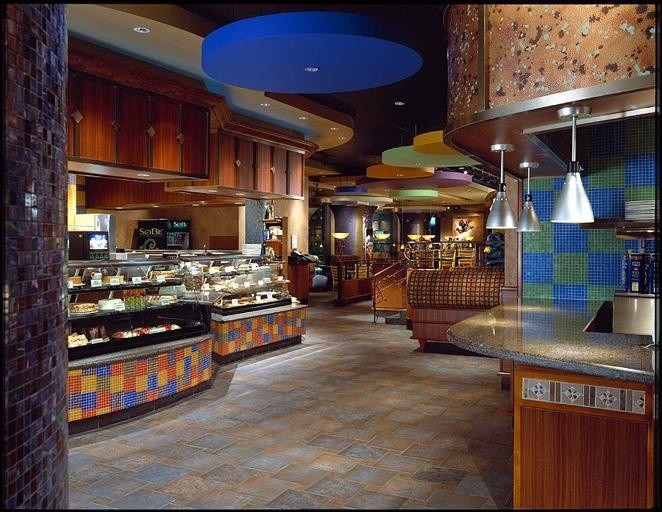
[68,276,183,320]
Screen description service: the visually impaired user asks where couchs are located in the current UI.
[408,266,505,351]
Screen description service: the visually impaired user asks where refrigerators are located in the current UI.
[136,218,191,250]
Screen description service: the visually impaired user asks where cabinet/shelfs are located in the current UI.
[68,67,306,204]
[67,260,215,435]
[190,255,308,364]
[261,216,289,290]
[335,260,394,305]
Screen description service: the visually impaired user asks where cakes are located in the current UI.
[67,271,182,348]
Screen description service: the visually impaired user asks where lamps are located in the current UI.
[486,144,518,230]
[408,234,421,240]
[515,162,541,232]
[422,234,435,240]
[551,105,595,223]
[375,233,390,259]
[331,233,349,255]
[443,234,475,242]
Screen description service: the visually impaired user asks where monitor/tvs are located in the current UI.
[69,231,110,259]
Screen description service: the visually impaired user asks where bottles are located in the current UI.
[263,226,278,240]
